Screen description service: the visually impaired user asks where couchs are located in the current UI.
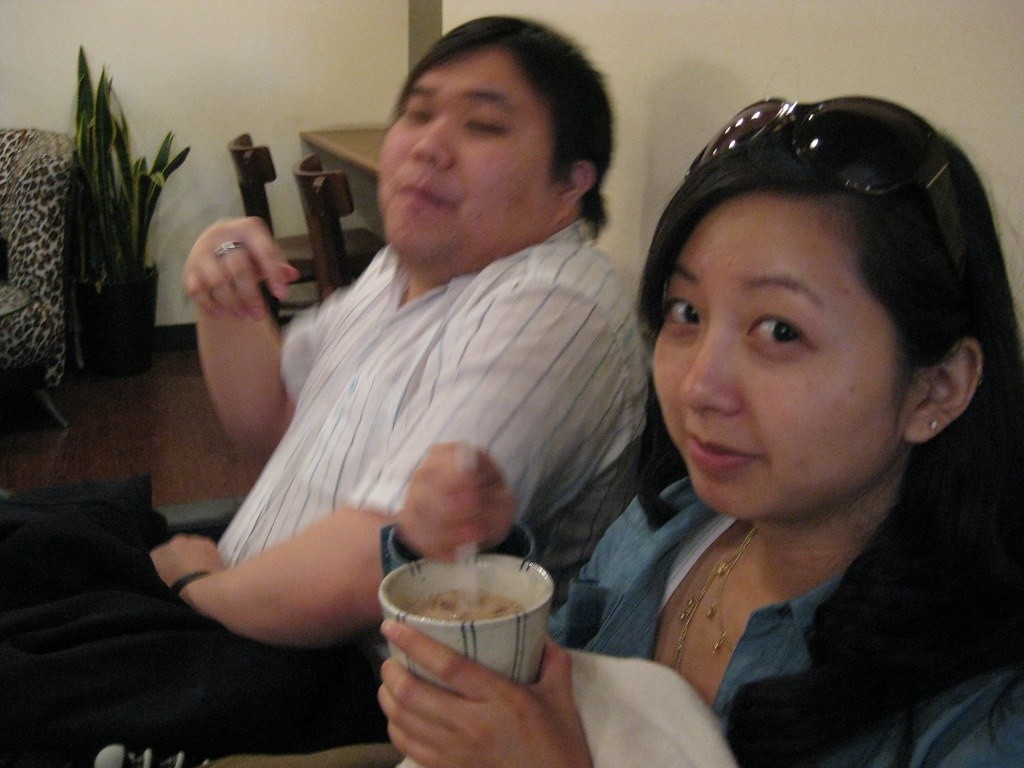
[0,130,84,430]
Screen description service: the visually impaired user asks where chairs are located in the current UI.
[230,134,388,327]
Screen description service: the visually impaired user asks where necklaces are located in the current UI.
[669,521,770,676]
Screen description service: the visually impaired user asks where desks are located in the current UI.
[300,127,390,179]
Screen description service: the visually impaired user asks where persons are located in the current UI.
[91,95,1022,768]
[0,13,654,768]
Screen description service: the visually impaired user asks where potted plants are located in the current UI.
[71,48,191,375]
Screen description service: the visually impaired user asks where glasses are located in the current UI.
[684,97,995,355]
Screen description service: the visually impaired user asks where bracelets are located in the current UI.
[167,569,219,597]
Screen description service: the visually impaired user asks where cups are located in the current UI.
[380,547,555,695]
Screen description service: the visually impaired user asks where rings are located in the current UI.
[213,239,249,256]
